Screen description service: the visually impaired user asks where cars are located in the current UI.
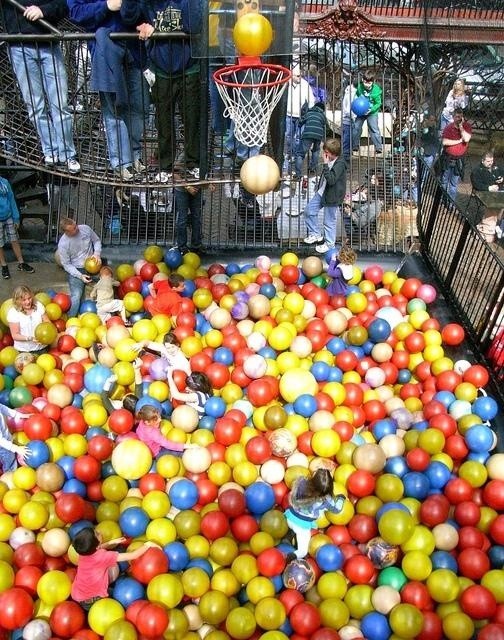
[374,40,504,117]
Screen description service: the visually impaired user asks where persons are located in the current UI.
[66,0,154,181]
[210,1,268,175]
[70,526,162,612]
[101,333,212,457]
[0,403,32,472]
[236,66,391,253]
[282,468,346,565]
[150,0,208,182]
[1,178,35,279]
[420,78,504,244]
[1,1,81,173]
[105,154,215,245]
[58,218,185,328]
[7,285,57,354]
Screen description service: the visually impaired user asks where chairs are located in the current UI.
[88,145,504,247]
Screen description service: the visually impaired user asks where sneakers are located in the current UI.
[304,234,335,253]
[116,159,207,183]
[44,154,82,173]
[1,266,10,279]
[179,244,213,256]
[17,263,35,273]
